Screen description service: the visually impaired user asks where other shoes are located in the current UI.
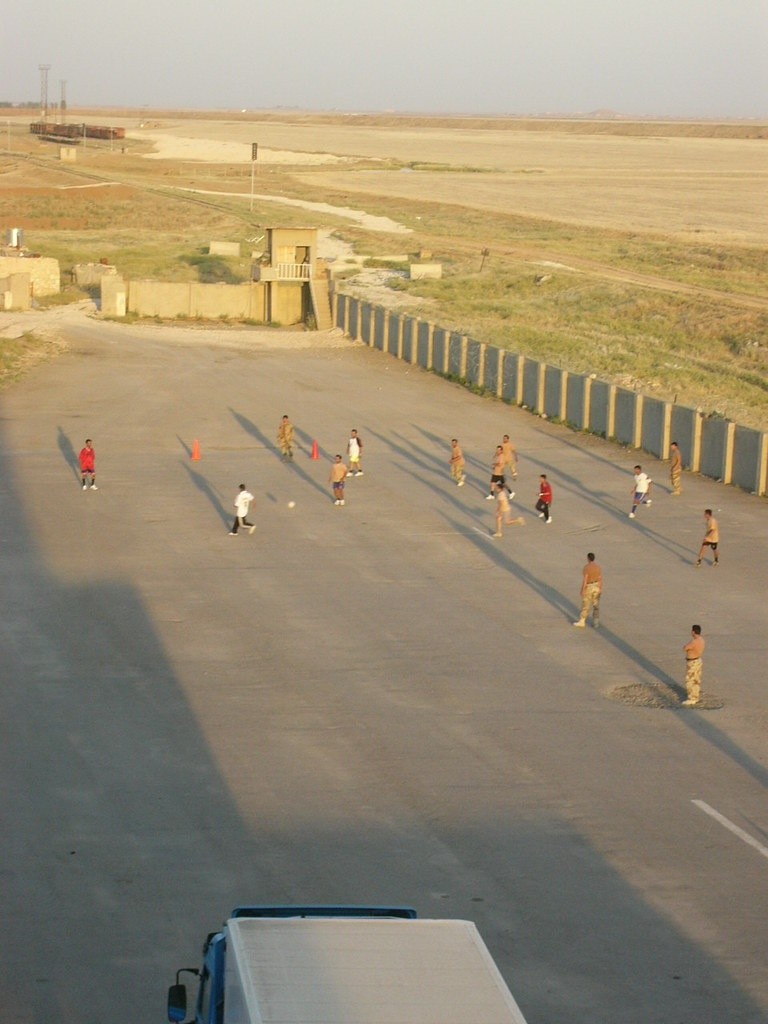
[509,492,515,500]
[518,517,526,527]
[538,513,544,517]
[346,473,352,478]
[682,699,700,704]
[228,532,238,535]
[573,620,585,627]
[354,472,364,476]
[457,482,464,486]
[486,495,495,499]
[83,485,87,490]
[493,533,502,537]
[463,475,466,482]
[334,500,340,505]
[670,491,680,496]
[646,500,652,507]
[90,485,98,490]
[694,562,701,567]
[340,500,345,505]
[249,526,255,534]
[546,517,552,523]
[628,512,635,518]
[711,561,719,569]
[594,623,599,628]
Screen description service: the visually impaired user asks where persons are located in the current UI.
[574,553,603,628]
[344,428,363,477]
[694,508,719,569]
[491,483,525,536]
[497,434,519,477]
[627,465,654,519]
[534,474,553,525]
[666,442,682,496]
[228,483,257,536]
[276,415,295,462]
[681,624,705,707]
[328,453,347,507]
[485,446,516,499]
[77,439,98,492]
[449,439,467,486]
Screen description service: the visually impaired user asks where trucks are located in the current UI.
[167,904,527,1024]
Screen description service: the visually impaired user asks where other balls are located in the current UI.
[289,500,296,508]
